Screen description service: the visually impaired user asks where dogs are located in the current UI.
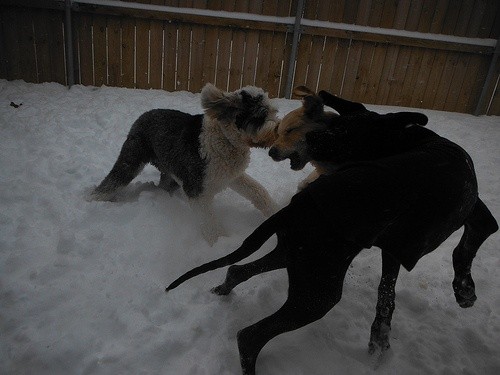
[165,91,499,375]
[84,83,279,248]
[268,86,341,195]
